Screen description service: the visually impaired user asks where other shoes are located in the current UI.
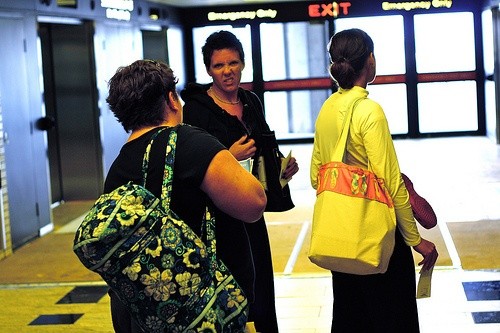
[399,172,438,230]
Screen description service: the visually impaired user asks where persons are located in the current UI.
[104,58,268,333]
[182,30,299,333]
[308,28,438,333]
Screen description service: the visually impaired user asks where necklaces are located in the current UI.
[210,87,240,104]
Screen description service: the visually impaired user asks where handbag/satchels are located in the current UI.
[72,126,250,333]
[306,94,398,277]
[241,87,296,214]
